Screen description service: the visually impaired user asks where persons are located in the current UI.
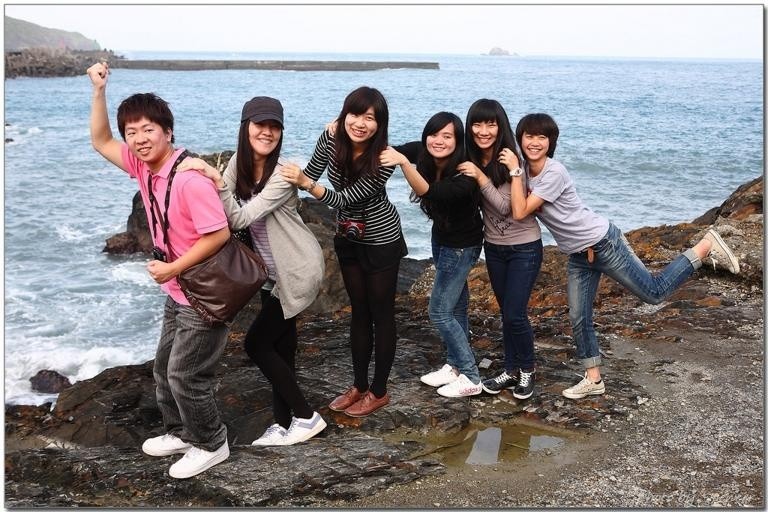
[498,113,740,400]
[175,96,328,445]
[279,87,407,419]
[456,98,542,400]
[325,111,484,399]
[85,61,231,480]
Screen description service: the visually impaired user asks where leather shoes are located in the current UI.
[345,391,390,417]
[329,386,369,411]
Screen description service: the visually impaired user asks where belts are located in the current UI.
[580,248,593,263]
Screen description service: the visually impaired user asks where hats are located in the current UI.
[242,96,284,127]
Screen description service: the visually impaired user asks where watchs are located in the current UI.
[509,166,523,177]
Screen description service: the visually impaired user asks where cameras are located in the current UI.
[151,246,167,263]
[337,220,365,242]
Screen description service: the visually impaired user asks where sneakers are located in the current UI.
[702,229,740,275]
[437,374,482,398]
[170,438,230,478]
[252,424,287,445]
[142,434,193,456]
[481,372,517,395]
[420,364,456,387]
[513,369,536,400]
[562,379,605,398]
[283,412,327,445]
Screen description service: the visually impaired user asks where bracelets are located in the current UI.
[306,179,315,193]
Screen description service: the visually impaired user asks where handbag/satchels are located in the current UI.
[177,235,269,327]
